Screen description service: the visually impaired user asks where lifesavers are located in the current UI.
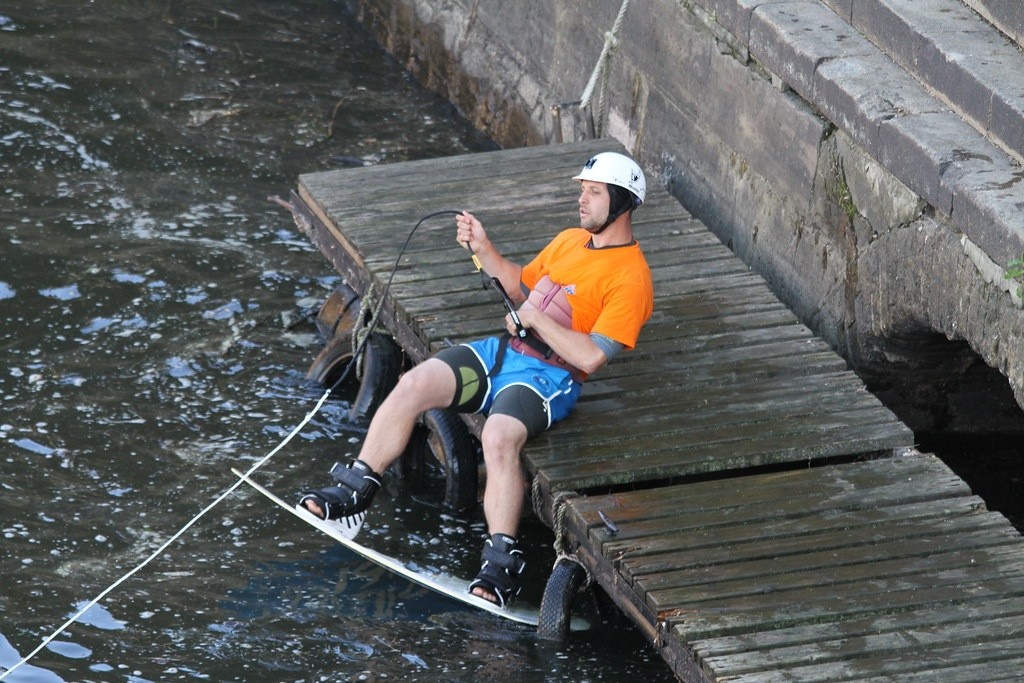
[305,327,400,417]
[393,408,479,514]
[536,560,596,641]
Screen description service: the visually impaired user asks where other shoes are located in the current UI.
[467,534,525,607]
[294,458,383,537]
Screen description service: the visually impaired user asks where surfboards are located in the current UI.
[227,463,594,632]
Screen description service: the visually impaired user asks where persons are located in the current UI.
[297,152,653,611]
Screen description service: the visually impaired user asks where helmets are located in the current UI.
[572,152,646,205]
